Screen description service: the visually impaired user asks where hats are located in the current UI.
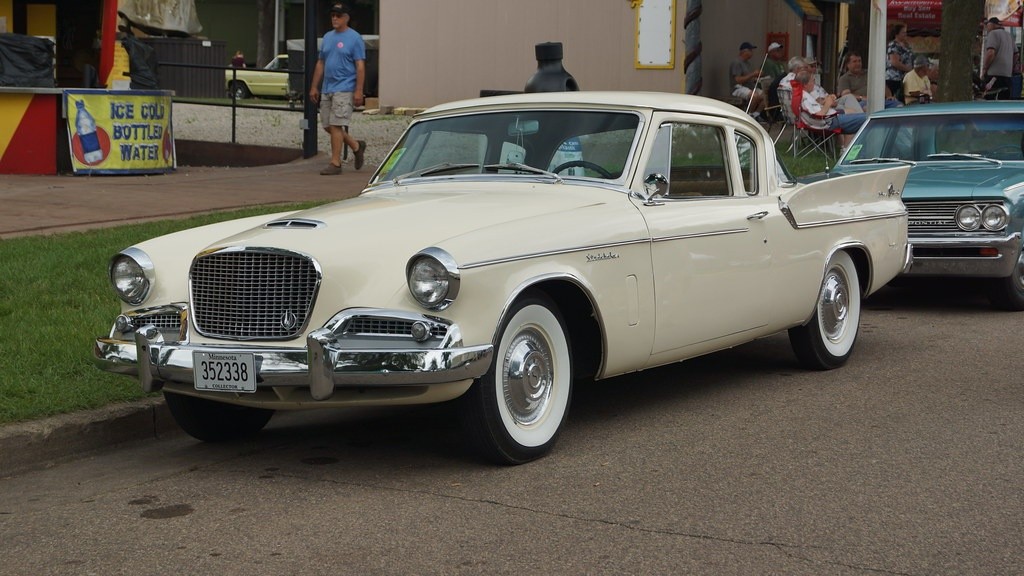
[984,17,1001,25]
[914,55,934,68]
[804,57,817,65]
[328,2,352,14]
[767,42,784,53]
[740,42,757,50]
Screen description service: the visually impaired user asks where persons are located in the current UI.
[762,42,785,74]
[902,55,933,102]
[778,56,857,155]
[730,42,764,123]
[310,4,365,175]
[805,57,816,72]
[795,73,867,133]
[839,54,904,108]
[983,17,1020,99]
[886,24,913,97]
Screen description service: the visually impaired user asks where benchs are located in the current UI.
[669,178,750,197]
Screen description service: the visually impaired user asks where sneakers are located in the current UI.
[320,162,342,175]
[353,140,367,170]
[839,147,847,157]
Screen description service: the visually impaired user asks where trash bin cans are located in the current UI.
[286,34,379,102]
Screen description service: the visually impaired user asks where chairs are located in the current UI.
[986,130,1023,157]
[934,128,972,153]
[759,76,842,162]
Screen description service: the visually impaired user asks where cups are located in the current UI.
[920,94,924,104]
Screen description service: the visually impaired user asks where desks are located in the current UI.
[0,84,173,173]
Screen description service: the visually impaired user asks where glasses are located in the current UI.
[331,13,349,18]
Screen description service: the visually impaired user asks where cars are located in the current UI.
[94,90,913,468]
[225,54,291,101]
[796,100,1024,313]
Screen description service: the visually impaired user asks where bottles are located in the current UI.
[75,99,103,164]
